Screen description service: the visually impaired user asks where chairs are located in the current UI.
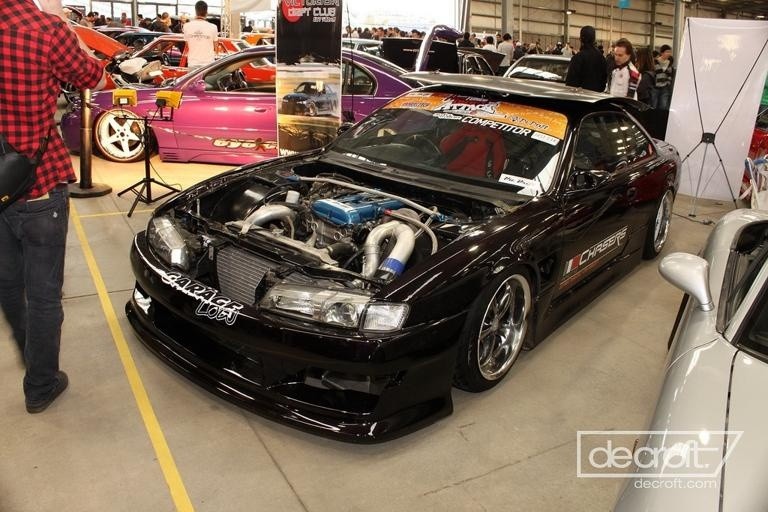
[439,124,507,183]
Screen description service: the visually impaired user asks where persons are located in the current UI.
[63,7,73,18]
[497,26,673,140]
[341,24,503,55]
[79,10,220,53]
[182,2,220,75]
[241,20,256,33]
[0,0,108,413]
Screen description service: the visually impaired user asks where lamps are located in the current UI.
[113,89,185,219]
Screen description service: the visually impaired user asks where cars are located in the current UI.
[124,72,676,446]
[610,207,768,511]
[61,24,640,167]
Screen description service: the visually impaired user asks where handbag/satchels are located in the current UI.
[1,132,39,212]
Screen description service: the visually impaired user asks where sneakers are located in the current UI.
[26,371,68,413]
